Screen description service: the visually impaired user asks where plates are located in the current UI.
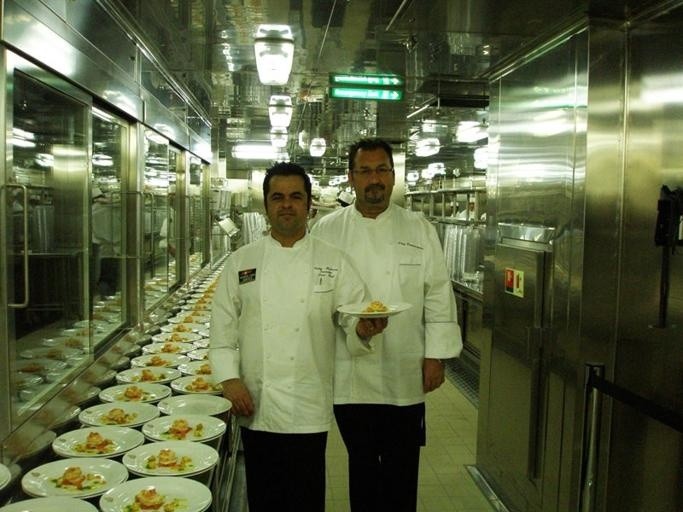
[0,250,234,512]
[337,302,411,317]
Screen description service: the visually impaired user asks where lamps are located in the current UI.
[254,37,326,157]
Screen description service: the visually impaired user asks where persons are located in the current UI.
[208,163,388,512]
[309,138,463,512]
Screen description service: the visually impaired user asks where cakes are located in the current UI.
[14,252,205,392]
[55,272,222,510]
[365,300,387,313]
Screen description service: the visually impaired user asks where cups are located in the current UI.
[444,225,479,272]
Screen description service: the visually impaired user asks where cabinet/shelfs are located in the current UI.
[2,40,215,466]
